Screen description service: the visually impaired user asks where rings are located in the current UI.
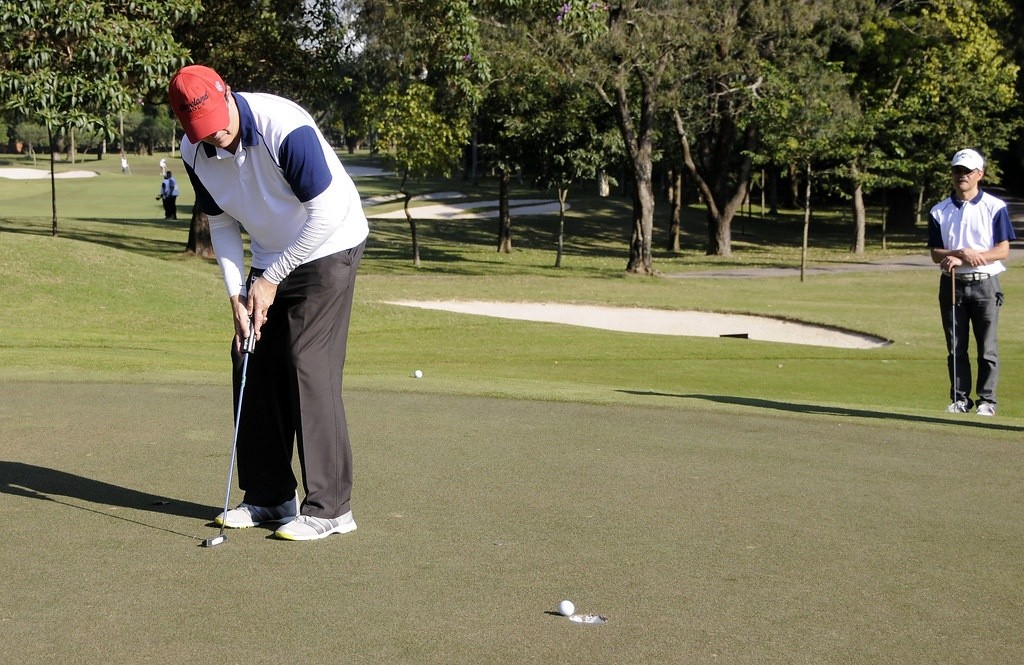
[262,317,267,324]
[946,263,949,265]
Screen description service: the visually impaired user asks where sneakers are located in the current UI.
[976,404,996,417]
[946,401,969,414]
[214,489,301,529]
[274,509,358,541]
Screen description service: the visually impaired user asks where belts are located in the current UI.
[939,269,991,283]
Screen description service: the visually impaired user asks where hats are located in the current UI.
[167,64,230,144]
[163,174,168,178]
[950,147,985,172]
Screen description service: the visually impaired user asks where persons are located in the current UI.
[168,66,369,542]
[928,149,1017,415]
[160,158,168,175]
[161,171,179,220]
[121,156,131,175]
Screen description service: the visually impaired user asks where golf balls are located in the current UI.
[414,368,423,378]
[557,599,576,617]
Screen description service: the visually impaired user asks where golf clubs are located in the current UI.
[200,277,259,548]
[954,268,958,412]
